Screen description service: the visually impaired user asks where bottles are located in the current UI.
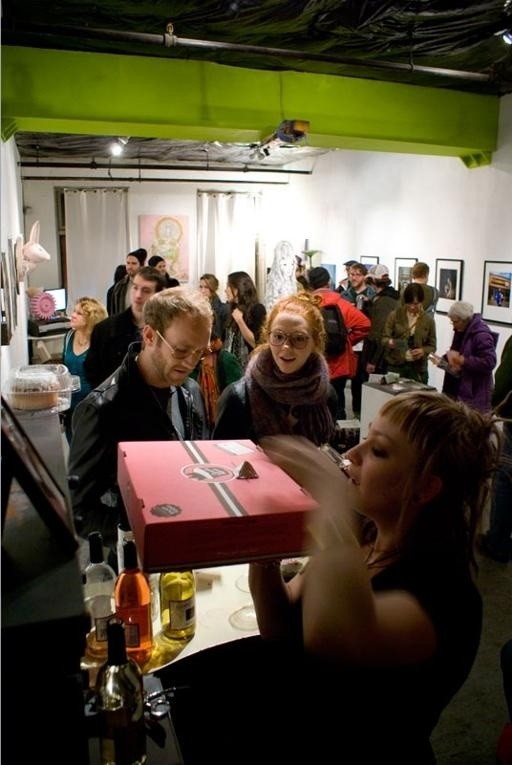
[81,530,118,631]
[159,554,197,640]
[95,615,148,765]
[428,353,456,375]
[114,542,153,666]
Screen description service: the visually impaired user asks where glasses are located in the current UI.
[156,330,213,361]
[268,328,308,349]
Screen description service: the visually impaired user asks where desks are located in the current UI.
[358,377,504,535]
[26,333,67,368]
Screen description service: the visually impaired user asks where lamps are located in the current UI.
[110,136,131,157]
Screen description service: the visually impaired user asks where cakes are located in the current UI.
[9,368,59,410]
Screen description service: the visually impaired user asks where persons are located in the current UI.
[442,302,499,413]
[299,258,440,417]
[60,248,339,567]
[491,335,512,430]
[258,388,504,764]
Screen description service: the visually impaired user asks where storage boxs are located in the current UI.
[115,437,327,575]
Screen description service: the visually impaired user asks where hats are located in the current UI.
[364,264,389,279]
[127,249,147,266]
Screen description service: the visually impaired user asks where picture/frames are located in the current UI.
[359,255,379,271]
[480,260,512,325]
[0,394,89,650]
[434,258,463,314]
[393,257,418,292]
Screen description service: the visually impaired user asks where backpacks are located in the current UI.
[320,304,346,354]
